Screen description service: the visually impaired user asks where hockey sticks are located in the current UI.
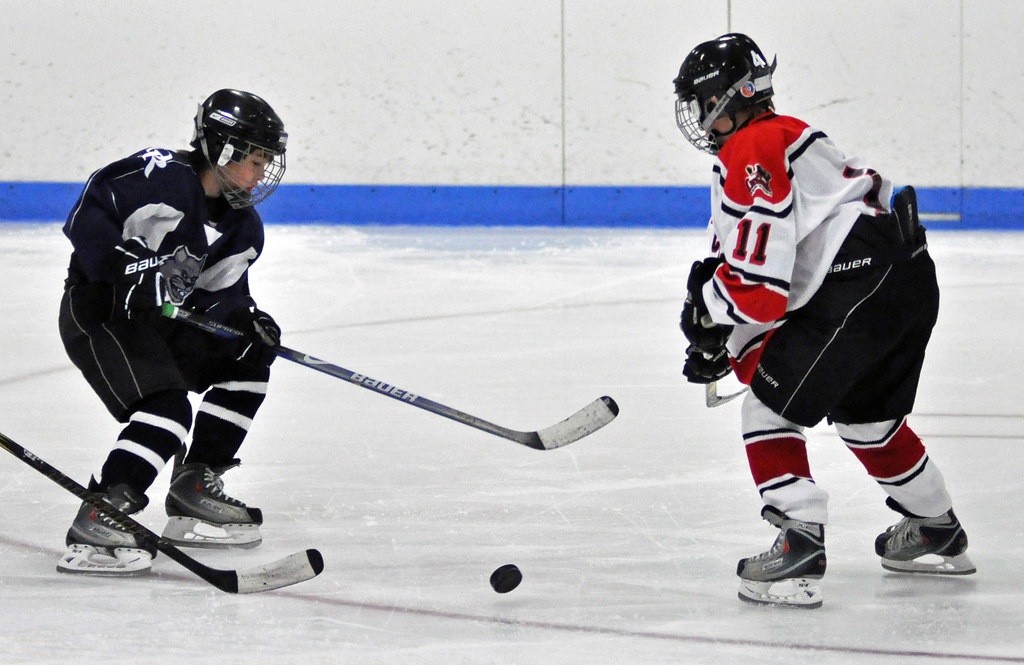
[701,315,751,408]
[161,300,620,450]
[0,433,325,595]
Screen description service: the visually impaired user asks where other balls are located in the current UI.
[489,563,523,594]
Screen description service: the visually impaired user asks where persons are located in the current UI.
[673,32,976,610]
[56,90,289,577]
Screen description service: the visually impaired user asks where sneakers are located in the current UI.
[161,442,263,544]
[737,504,827,610]
[875,496,977,575]
[56,474,158,572]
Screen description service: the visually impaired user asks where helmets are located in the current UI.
[672,32,775,155]
[189,88,289,209]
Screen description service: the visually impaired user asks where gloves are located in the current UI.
[679,252,735,355]
[221,305,283,368]
[100,236,167,322]
[681,343,734,384]
